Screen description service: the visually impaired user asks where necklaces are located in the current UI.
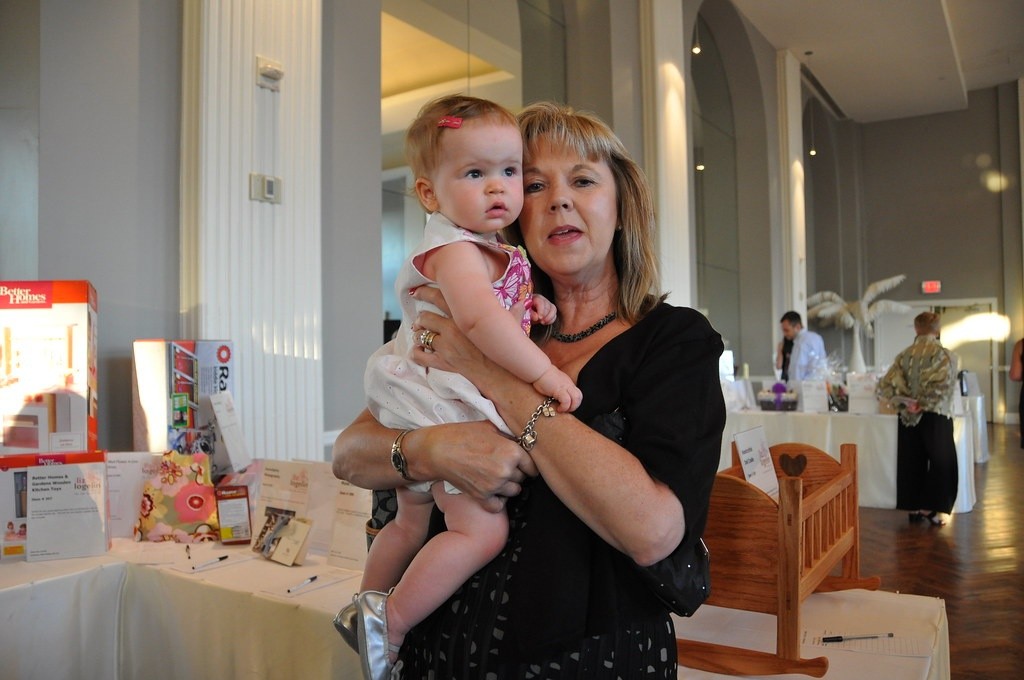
[549,311,616,343]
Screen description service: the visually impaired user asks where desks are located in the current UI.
[717,396,989,514]
[0,538,950,680]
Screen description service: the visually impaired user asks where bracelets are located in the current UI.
[515,396,557,451]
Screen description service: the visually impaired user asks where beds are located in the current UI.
[675,441,882,679]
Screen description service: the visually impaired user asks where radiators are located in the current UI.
[178,0,325,462]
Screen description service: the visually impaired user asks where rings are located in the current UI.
[418,331,439,352]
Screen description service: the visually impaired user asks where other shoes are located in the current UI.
[334,599,358,653]
[353,588,403,680]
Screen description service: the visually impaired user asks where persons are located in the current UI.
[334,94,583,680]
[333,102,728,680]
[775,311,829,388]
[877,312,958,527]
[1008,338,1024,448]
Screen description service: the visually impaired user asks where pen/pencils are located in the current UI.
[191,554,229,570]
[822,631,894,642]
[288,573,317,593]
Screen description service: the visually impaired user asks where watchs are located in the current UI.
[390,429,419,484]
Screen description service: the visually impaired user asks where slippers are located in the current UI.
[919,509,946,526]
[909,514,924,522]
